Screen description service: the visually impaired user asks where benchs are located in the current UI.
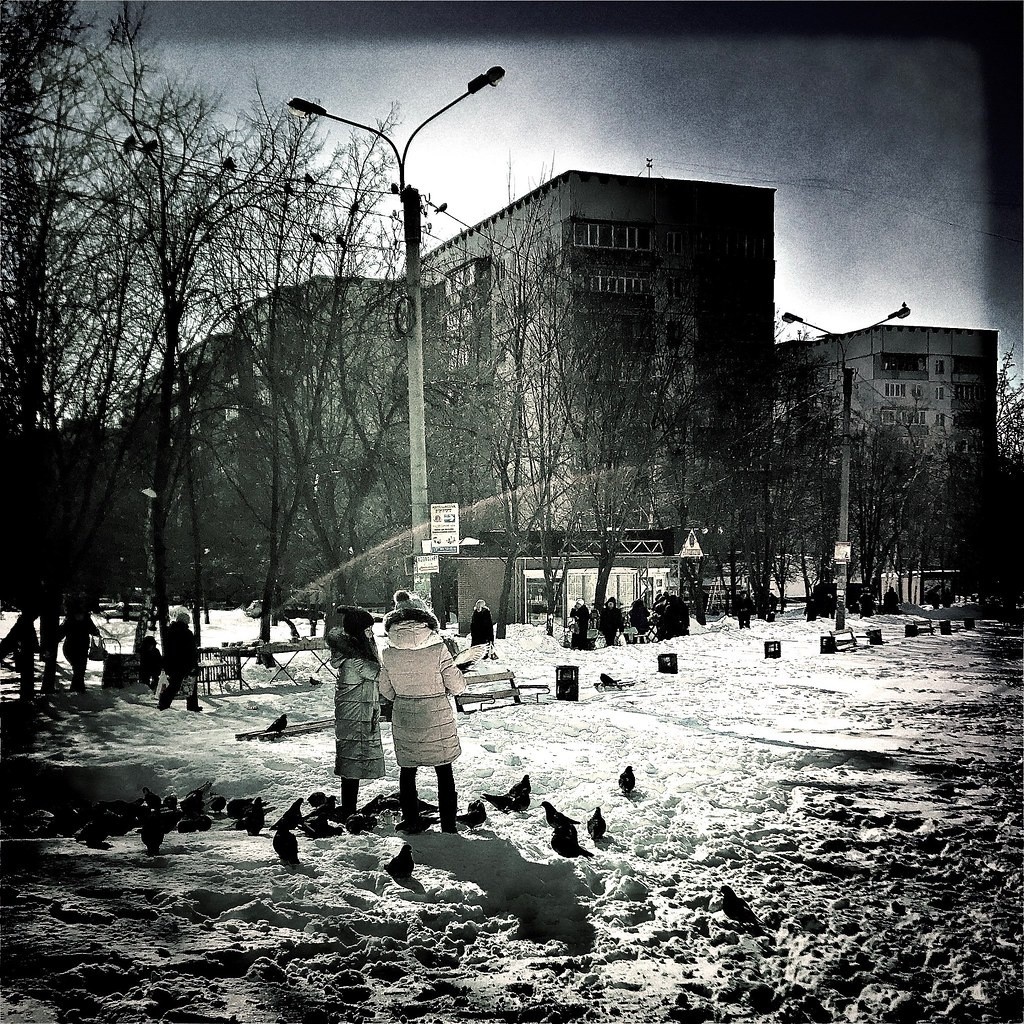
[913,619,936,636]
[454,669,551,715]
[829,627,859,652]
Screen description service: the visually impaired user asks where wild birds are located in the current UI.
[48,779,442,886]
[600,673,622,690]
[309,677,323,687]
[480,774,531,814]
[265,714,288,742]
[455,799,486,832]
[618,765,636,796]
[442,637,490,676]
[587,807,607,843]
[539,801,595,860]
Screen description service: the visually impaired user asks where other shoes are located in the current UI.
[187,706,203,712]
[158,704,171,711]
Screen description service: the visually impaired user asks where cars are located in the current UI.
[245,600,263,618]
[98,587,158,623]
[675,552,888,589]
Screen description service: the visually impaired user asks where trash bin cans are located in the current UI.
[100,652,140,691]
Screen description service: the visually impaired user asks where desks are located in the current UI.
[214,646,337,691]
[646,623,659,642]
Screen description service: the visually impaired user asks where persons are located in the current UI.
[470,600,494,646]
[942,588,953,608]
[629,599,650,636]
[931,592,940,610]
[652,592,690,640]
[570,598,589,650]
[734,591,755,628]
[802,589,835,621]
[141,636,162,692]
[157,612,203,712]
[58,601,100,692]
[597,596,624,648]
[379,591,467,834]
[884,587,900,615]
[860,594,876,618]
[327,605,386,818]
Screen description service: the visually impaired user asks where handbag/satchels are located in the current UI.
[90,635,109,661]
[179,669,196,697]
[618,635,626,646]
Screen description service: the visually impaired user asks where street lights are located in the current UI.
[782,307,911,631]
[286,65,508,610]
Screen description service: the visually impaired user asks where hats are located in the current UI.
[576,599,584,606]
[740,587,746,593]
[658,596,666,602]
[337,605,374,631]
